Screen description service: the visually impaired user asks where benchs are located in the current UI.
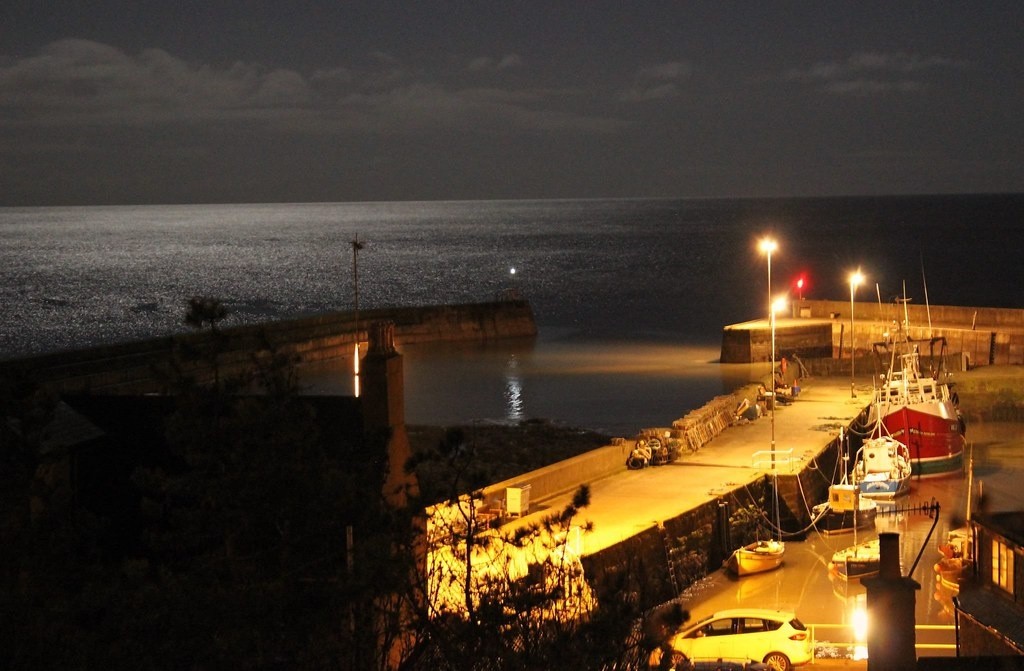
[429,504,504,551]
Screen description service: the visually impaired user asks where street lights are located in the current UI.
[849,272,864,400]
[756,232,783,538]
[350,231,364,346]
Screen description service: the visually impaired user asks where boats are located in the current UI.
[830,540,886,583]
[728,539,788,577]
[848,382,913,500]
[932,556,975,593]
[810,427,879,534]
[865,278,969,480]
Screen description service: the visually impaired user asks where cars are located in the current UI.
[659,609,817,671]
